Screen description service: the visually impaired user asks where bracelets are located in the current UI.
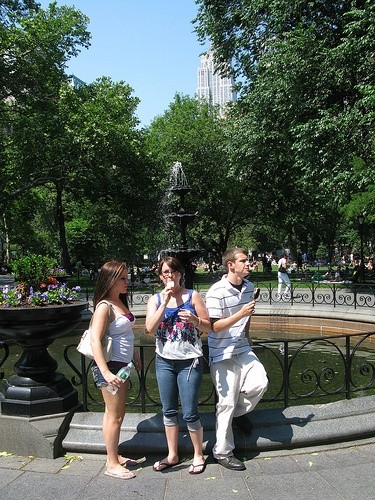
[198,317,201,325]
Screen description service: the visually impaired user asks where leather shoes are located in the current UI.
[213,455,246,471]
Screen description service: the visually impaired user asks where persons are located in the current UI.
[322,253,373,281]
[205,247,269,471]
[145,257,211,475]
[90,260,137,480]
[277,253,291,299]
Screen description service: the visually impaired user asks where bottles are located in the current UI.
[107,362,133,395]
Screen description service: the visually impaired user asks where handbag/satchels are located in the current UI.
[76,300,113,363]
[278,264,286,273]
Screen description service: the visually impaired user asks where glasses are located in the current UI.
[161,270,178,276]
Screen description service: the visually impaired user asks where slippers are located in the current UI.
[152,460,179,471]
[104,469,136,480]
[106,458,138,468]
[188,460,207,474]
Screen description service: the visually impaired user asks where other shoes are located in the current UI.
[285,296,291,299]
